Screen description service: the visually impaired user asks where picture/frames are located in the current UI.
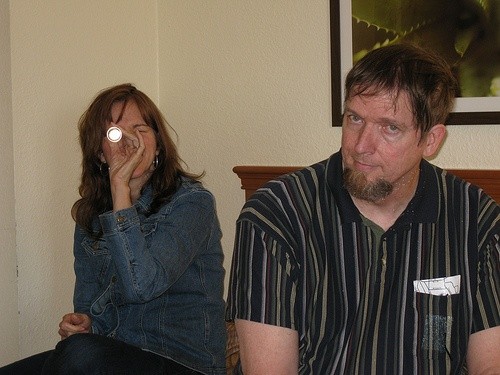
[330,0,500,127]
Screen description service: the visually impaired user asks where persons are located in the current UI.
[0,82,226,375]
[224,40,500,375]
